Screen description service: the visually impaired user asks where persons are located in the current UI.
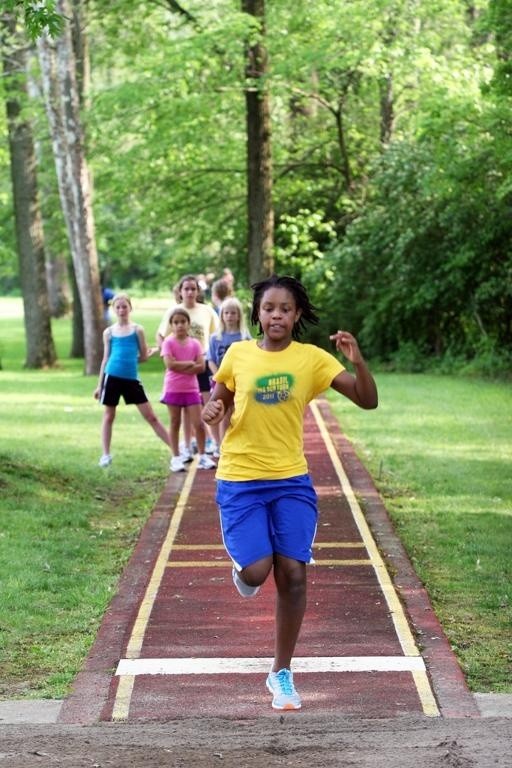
[92,264,253,474]
[200,274,379,713]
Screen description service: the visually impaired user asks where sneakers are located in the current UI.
[232,565,260,599]
[195,438,221,472]
[97,453,114,471]
[169,436,197,474]
[264,661,303,715]
